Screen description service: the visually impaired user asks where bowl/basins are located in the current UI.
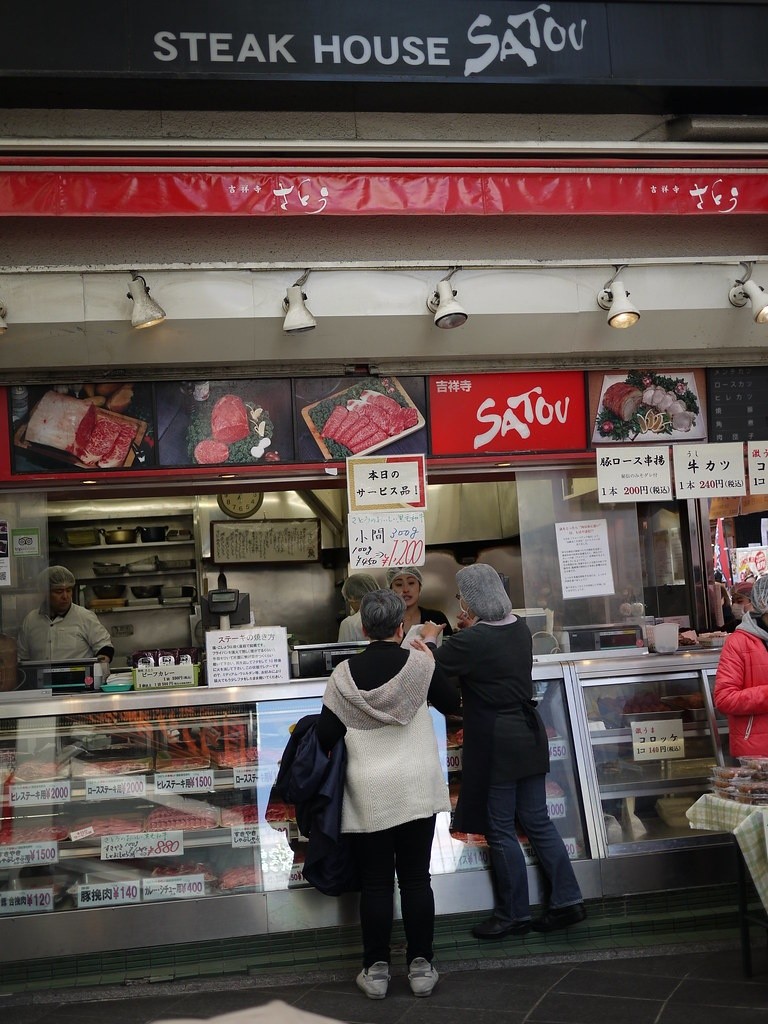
[92,585,126,599]
[130,585,165,598]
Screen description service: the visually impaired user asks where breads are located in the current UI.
[81,383,134,414]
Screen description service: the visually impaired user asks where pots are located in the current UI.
[137,526,170,542]
[99,526,141,544]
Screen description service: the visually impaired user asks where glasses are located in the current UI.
[455,594,460,600]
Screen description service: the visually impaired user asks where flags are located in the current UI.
[713,518,731,593]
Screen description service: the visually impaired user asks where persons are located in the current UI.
[337,566,452,643]
[420,563,587,940]
[316,587,452,999]
[16,565,115,663]
[678,572,768,765]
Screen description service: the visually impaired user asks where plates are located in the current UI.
[591,371,708,444]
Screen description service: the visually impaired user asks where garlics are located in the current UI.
[52,384,83,398]
[251,437,272,458]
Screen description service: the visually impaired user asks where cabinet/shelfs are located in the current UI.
[18,508,199,616]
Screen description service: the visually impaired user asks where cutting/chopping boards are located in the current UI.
[12,390,148,470]
[301,369,428,465]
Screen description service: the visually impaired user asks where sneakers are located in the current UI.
[408,958,439,997]
[471,915,531,939]
[356,961,391,999]
[535,904,588,930]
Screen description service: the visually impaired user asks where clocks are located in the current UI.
[217,492,264,519]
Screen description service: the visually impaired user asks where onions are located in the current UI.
[265,451,280,462]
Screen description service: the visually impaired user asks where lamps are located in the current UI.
[728,279,768,324]
[427,281,467,329]
[126,271,166,329]
[598,282,639,329]
[283,286,317,333]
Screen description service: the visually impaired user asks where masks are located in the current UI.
[731,603,753,620]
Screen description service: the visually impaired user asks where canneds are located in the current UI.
[11,386,29,420]
